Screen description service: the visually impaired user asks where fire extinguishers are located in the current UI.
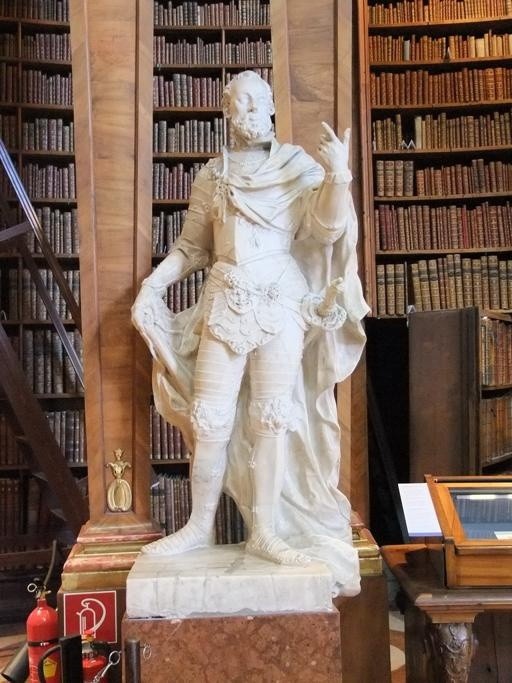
[27,577,61,683]
[81,639,112,683]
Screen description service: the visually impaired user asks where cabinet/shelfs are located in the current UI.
[409,307,512,478]
[363,2,512,316]
[150,22,272,467]
[0,14,89,565]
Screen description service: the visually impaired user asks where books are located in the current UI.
[153,1,271,65]
[367,1,511,62]
[374,159,511,252]
[2,63,74,152]
[153,68,273,153]
[1,0,72,62]
[149,405,191,460]
[1,408,85,553]
[482,316,512,461]
[1,161,80,254]
[152,267,203,313]
[152,162,204,257]
[150,472,248,547]
[371,69,511,151]
[377,255,512,317]
[1,564,65,571]
[9,269,84,394]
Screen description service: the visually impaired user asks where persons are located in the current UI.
[129,71,370,568]
